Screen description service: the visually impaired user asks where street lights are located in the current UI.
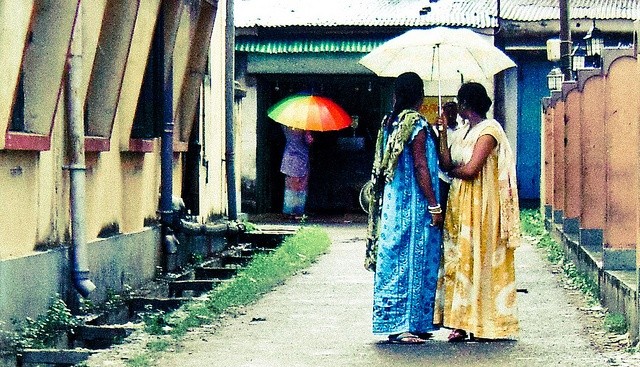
[545,38,573,81]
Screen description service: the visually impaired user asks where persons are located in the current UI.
[365,71,444,344]
[433,81,522,343]
[433,101,464,147]
[280,123,315,220]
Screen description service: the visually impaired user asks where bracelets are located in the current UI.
[427,204,443,215]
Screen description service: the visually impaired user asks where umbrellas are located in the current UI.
[355,24,519,132]
[266,92,353,133]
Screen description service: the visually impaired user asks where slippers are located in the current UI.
[389,333,426,344]
[448,331,468,342]
